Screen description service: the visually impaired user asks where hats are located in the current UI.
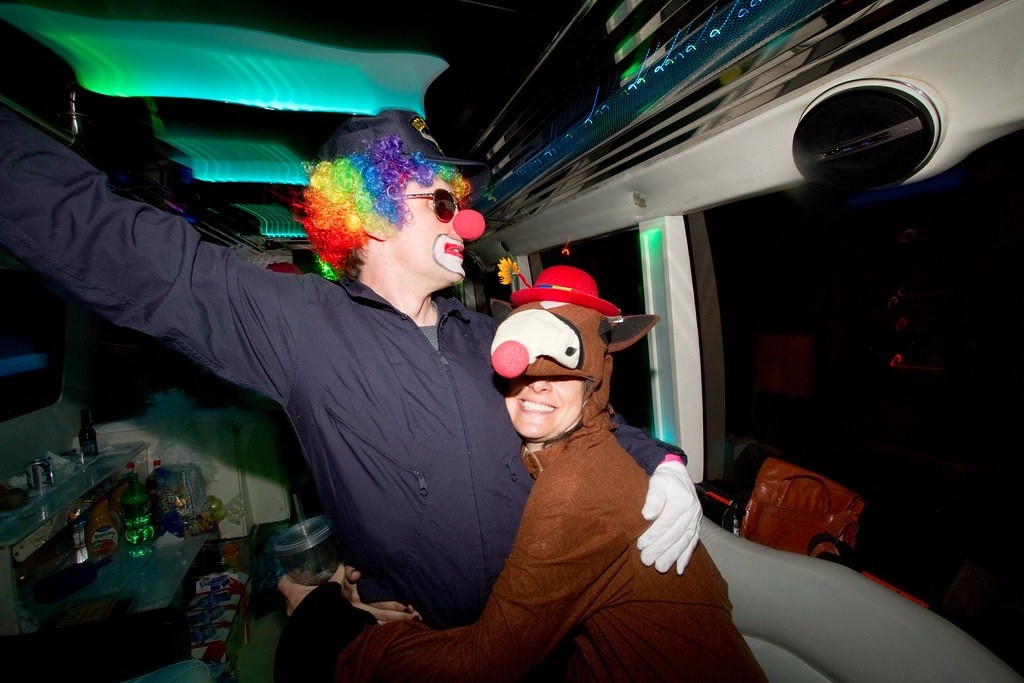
[319,109,490,211]
[511,265,621,315]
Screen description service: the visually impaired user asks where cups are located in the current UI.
[274,513,338,589]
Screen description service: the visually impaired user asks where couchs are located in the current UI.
[699,516,1024,683]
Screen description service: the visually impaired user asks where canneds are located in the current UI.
[25,455,54,493]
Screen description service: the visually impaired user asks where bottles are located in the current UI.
[122,473,154,545]
[85,498,120,556]
[123,463,135,474]
[79,408,99,457]
[151,459,161,468]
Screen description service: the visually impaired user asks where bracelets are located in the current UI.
[660,454,683,464]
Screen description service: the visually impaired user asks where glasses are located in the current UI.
[405,188,468,223]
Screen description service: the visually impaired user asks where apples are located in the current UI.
[8,488,27,508]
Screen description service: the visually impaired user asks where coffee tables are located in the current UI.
[0,442,223,683]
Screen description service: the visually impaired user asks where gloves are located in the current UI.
[635,458,703,575]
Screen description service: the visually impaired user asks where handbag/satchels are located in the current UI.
[741,457,864,558]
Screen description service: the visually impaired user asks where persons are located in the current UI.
[0,106,706,629]
[275,259,770,682]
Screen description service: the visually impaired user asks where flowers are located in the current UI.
[495,258,534,290]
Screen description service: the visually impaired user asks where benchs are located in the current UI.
[175,570,255,683]
